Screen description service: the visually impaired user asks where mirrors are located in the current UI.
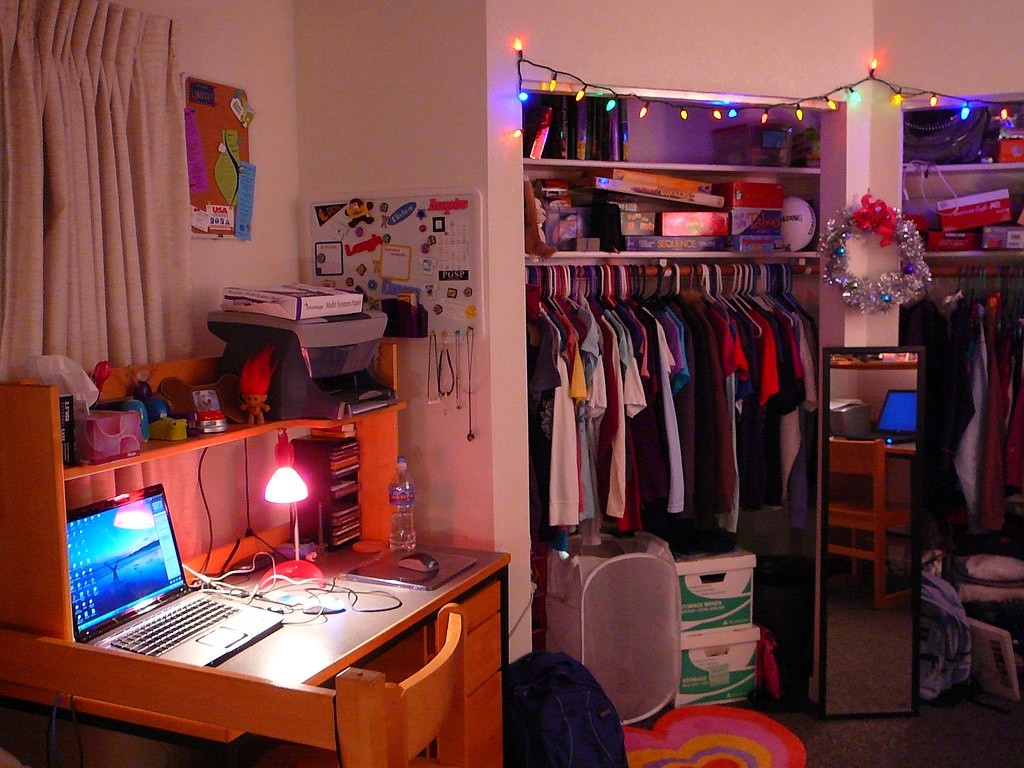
[816,345,929,722]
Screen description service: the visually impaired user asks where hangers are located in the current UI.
[955,261,1024,344]
[525,260,817,339]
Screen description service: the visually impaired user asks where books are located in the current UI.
[311,423,361,547]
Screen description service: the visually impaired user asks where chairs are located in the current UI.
[829,435,911,612]
[335,603,470,768]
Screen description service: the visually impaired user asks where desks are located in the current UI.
[829,436,917,459]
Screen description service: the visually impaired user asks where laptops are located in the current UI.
[65,482,285,663]
[846,389,918,443]
[340,547,477,592]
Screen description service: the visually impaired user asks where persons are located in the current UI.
[240,351,270,425]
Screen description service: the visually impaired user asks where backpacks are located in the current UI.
[918,570,972,703]
[502,650,629,768]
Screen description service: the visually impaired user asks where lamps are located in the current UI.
[258,427,326,591]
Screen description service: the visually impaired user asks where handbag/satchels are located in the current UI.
[902,107,993,165]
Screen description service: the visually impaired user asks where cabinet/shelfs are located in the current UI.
[519,80,839,259]
[1,344,513,768]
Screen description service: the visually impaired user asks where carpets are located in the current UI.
[622,703,808,768]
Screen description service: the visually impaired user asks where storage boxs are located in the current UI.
[544,206,591,251]
[221,283,366,321]
[674,546,756,637]
[58,394,77,467]
[985,138,1024,163]
[981,225,1024,250]
[937,188,1012,232]
[710,118,795,167]
[672,623,761,709]
[581,168,787,254]
[927,230,982,251]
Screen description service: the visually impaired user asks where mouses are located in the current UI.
[398,552,439,573]
[302,596,347,614]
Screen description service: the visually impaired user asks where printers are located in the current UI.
[207,308,398,423]
[828,404,873,436]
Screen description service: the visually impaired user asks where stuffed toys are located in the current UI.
[523,170,557,261]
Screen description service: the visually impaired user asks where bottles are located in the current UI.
[387,456,417,553]
